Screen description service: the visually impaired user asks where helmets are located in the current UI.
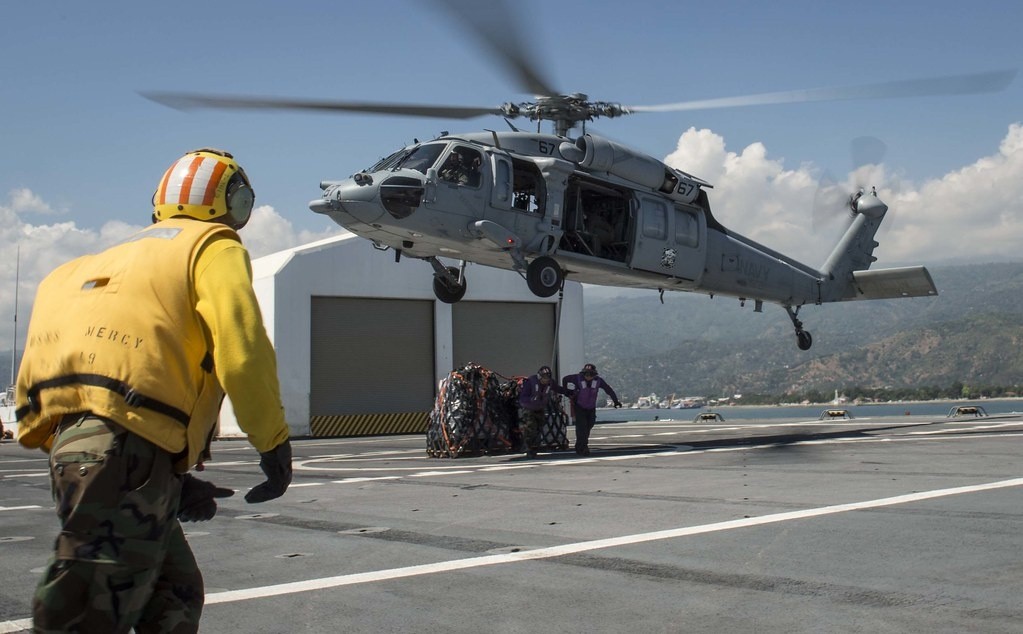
[584,364,596,373]
[451,146,464,161]
[152,148,254,229]
[538,366,551,381]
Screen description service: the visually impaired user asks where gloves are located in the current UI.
[178,474,234,522]
[244,438,292,503]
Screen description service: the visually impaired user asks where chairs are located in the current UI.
[568,204,628,261]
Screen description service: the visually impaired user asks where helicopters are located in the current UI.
[134,1,1017,352]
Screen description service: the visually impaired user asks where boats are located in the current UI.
[631,391,705,410]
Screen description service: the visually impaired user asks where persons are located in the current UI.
[441,154,470,185]
[14,149,293,634]
[516,366,574,458]
[562,364,622,455]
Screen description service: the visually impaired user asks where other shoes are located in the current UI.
[575,444,589,455]
[527,449,536,459]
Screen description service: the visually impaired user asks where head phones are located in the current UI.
[152,174,253,228]
[580,368,598,378]
[536,369,552,380]
